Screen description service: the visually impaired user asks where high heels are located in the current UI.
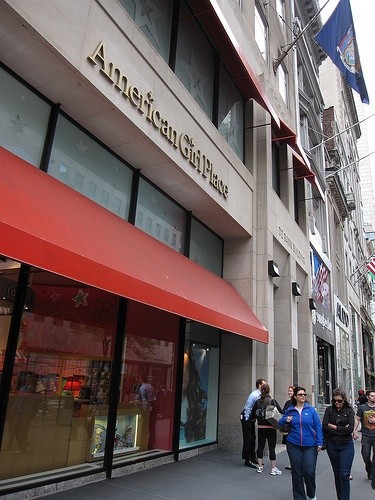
[2,440,13,450]
[15,440,30,454]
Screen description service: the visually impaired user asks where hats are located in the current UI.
[357,390,364,395]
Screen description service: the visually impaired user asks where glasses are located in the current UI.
[332,399,344,403]
[298,394,307,396]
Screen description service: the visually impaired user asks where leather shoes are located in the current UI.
[244,461,259,469]
[251,457,264,465]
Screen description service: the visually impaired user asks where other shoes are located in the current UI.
[72,410,80,417]
[285,466,291,469]
[349,475,353,479]
[368,472,373,480]
[371,475,375,490]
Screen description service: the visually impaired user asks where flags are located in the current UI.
[312,0,369,105]
[366,257,375,281]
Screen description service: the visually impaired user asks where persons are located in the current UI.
[355,390,368,432]
[352,391,375,491]
[67,376,94,443]
[321,389,355,500]
[3,370,40,454]
[179,343,206,444]
[138,374,157,450]
[240,378,267,469]
[248,383,283,476]
[283,385,309,470]
[279,386,324,500]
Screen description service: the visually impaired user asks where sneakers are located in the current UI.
[257,467,263,472]
[309,490,316,500]
[271,467,282,475]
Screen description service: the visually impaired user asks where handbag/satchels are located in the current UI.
[146,386,157,402]
[321,406,333,450]
[266,399,284,429]
